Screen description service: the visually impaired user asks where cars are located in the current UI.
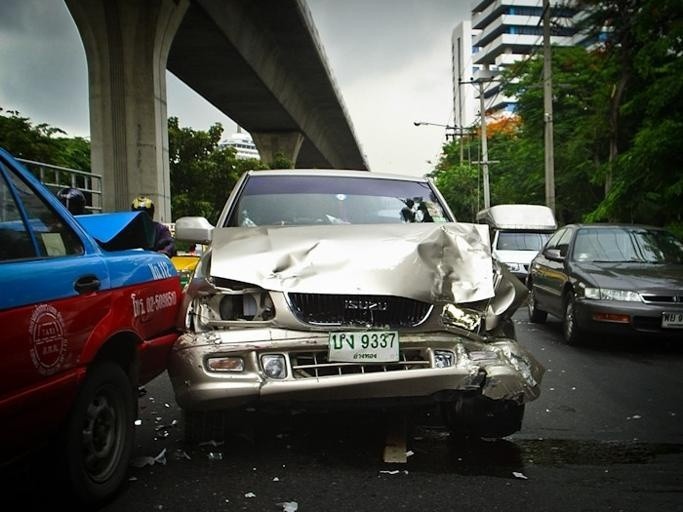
[0,148,185,502]
[155,222,210,290]
[523,222,682,346]
[166,165,546,443]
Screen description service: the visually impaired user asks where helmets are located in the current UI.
[57,187,86,214]
[131,196,154,220]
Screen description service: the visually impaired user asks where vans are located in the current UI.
[474,203,557,278]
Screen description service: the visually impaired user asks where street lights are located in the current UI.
[412,120,480,225]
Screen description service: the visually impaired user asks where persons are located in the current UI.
[130,197,175,259]
[55,186,91,216]
